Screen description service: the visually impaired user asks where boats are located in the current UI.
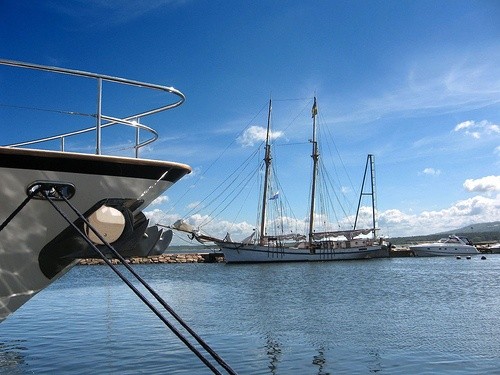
[407,233,483,258]
[0,59,192,324]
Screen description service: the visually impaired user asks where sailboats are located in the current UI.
[155,88,392,264]
